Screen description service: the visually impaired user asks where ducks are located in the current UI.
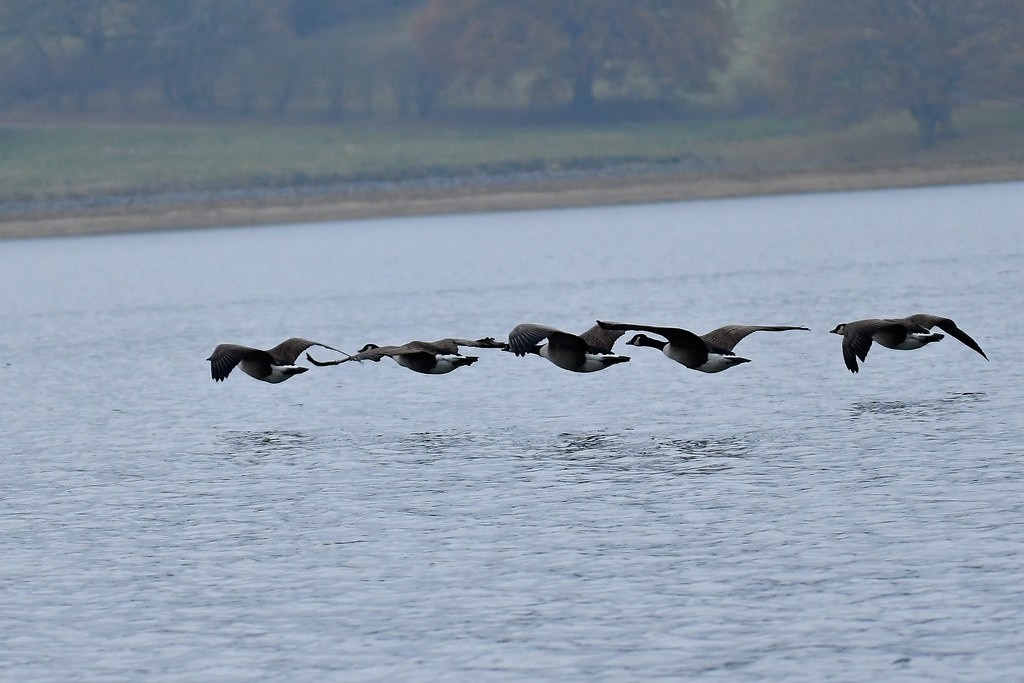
[204,308,991,387]
